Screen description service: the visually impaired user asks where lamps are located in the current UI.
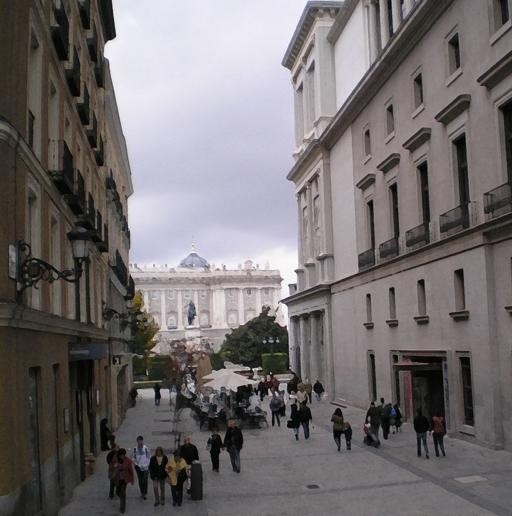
[7,214,96,293]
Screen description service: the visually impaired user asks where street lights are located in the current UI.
[262,336,280,375]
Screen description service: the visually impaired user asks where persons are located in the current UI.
[342,422,352,450]
[100,418,115,451]
[429,416,446,457]
[331,408,344,452]
[224,419,243,473]
[129,387,138,407]
[106,435,190,514]
[153,383,161,406]
[179,435,199,465]
[258,372,325,441]
[363,397,403,448]
[413,408,430,459]
[206,430,224,472]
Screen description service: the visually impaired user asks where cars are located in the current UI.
[132,351,161,360]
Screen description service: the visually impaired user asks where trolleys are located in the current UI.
[362,417,378,446]
